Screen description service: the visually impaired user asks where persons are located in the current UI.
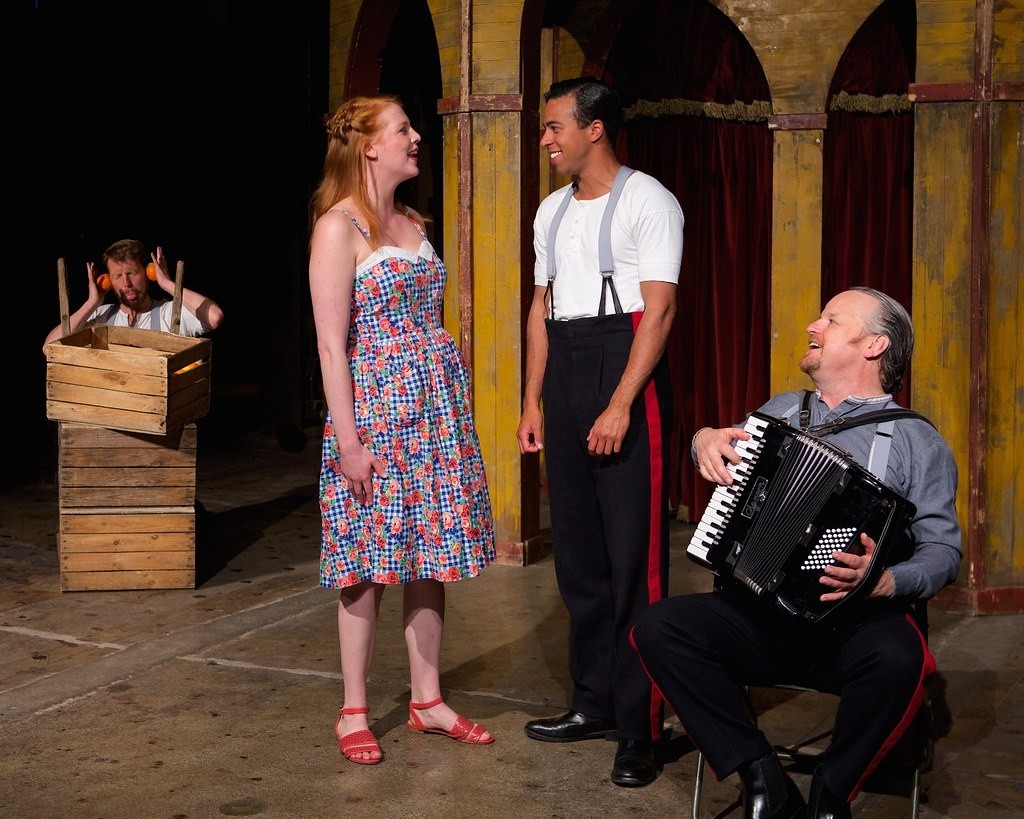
[630,285,963,819]
[307,96,498,764]
[40,238,223,359]
[522,77,685,786]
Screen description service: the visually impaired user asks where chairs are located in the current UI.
[690,576,929,819]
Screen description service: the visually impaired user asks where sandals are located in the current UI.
[334,706,382,764]
[406,695,494,744]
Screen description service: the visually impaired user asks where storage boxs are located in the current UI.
[57,420,198,509]
[46,322,213,438]
[56,508,196,593]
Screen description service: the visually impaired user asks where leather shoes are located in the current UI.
[807,762,852,819]
[611,715,657,786]
[739,746,808,819]
[524,709,621,742]
